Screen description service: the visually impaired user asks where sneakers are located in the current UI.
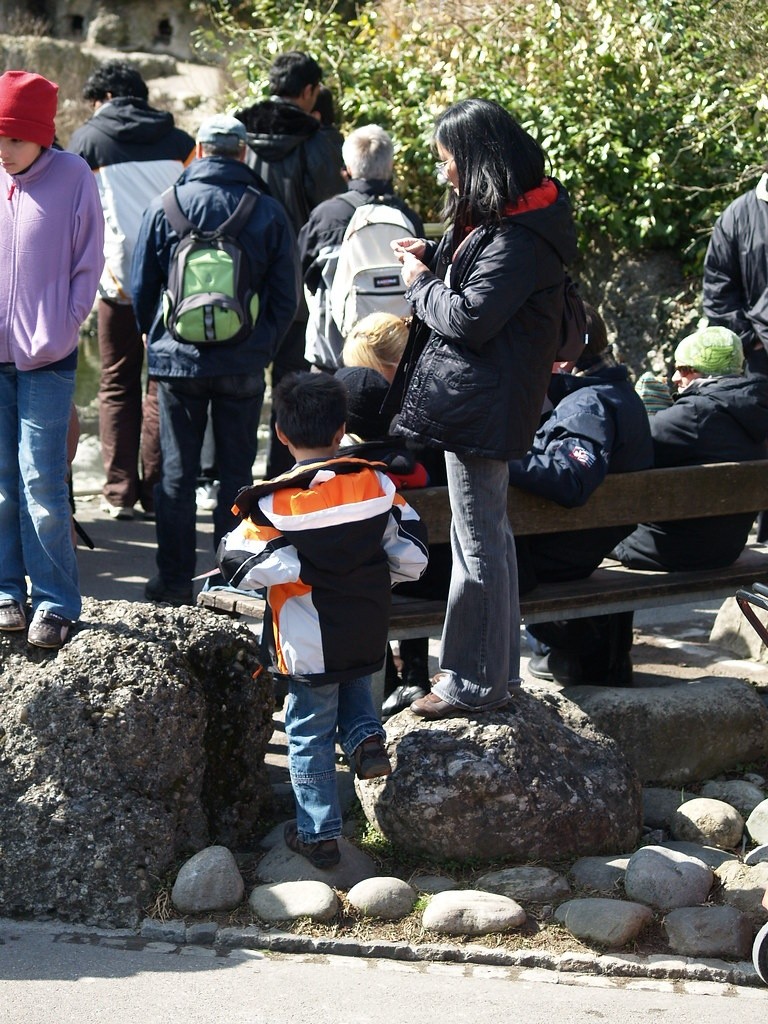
[354,742,391,780]
[100,494,135,518]
[1,598,71,647]
[284,821,342,870]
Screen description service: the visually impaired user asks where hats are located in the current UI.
[196,114,248,147]
[334,366,392,434]
[0,70,59,148]
[675,326,745,376]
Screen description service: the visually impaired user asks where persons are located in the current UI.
[531,326,768,685]
[700,167,768,530]
[300,126,431,433]
[194,53,343,495]
[379,100,587,718]
[128,111,309,612]
[68,65,203,525]
[388,292,654,712]
[213,375,428,870]
[0,69,105,650]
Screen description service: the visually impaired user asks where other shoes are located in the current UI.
[145,573,194,606]
[411,673,520,718]
[383,671,429,719]
[528,652,634,685]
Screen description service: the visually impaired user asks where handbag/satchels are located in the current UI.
[557,274,585,363]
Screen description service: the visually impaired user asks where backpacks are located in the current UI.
[304,191,421,339]
[160,184,261,346]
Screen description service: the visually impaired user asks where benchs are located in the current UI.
[193,456,768,724]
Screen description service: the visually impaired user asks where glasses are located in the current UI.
[433,156,457,172]
[678,367,698,378]
